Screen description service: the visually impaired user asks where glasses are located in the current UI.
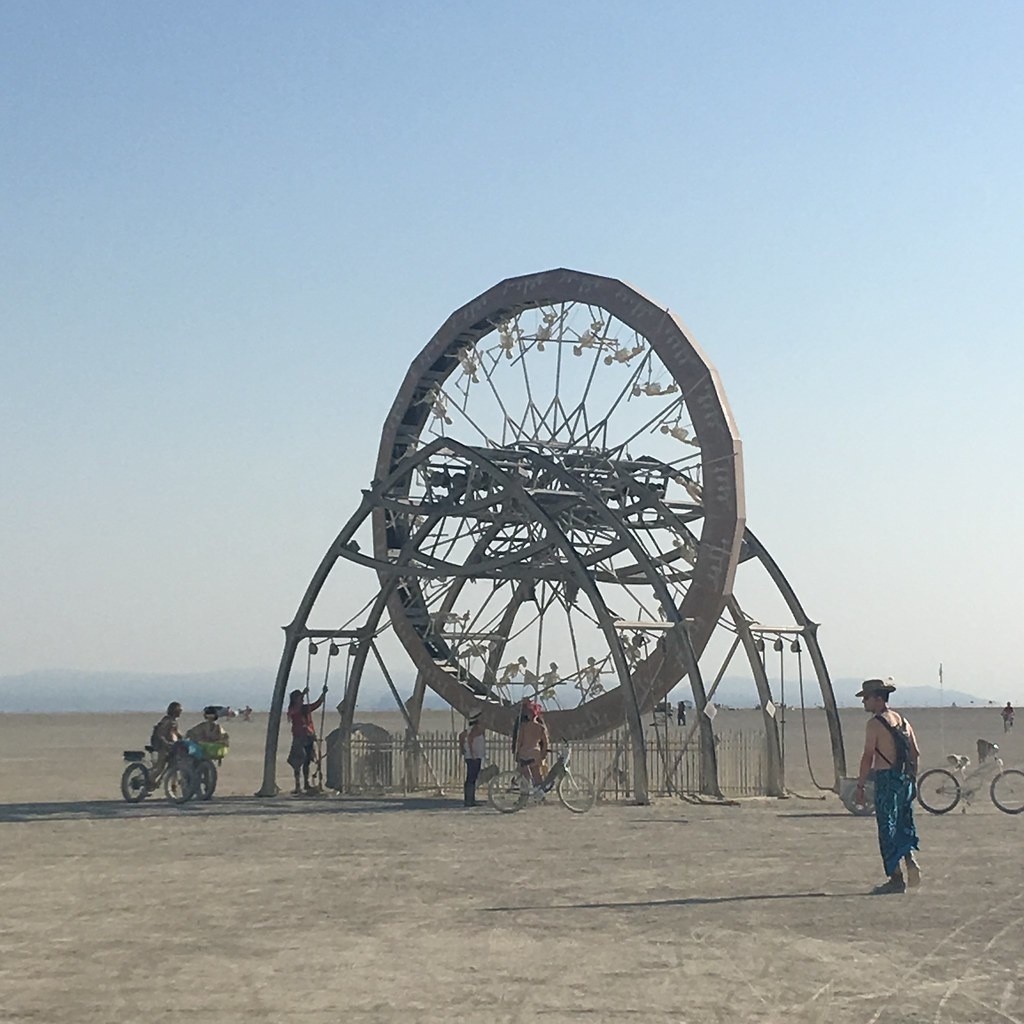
[864,695,878,701]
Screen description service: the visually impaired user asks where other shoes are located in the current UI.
[873,873,906,892]
[148,774,156,783]
[906,861,922,887]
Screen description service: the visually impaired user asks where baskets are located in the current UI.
[175,754,196,766]
[202,743,225,759]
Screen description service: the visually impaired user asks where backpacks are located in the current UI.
[875,714,911,781]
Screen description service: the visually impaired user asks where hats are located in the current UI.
[469,710,484,722]
[519,697,533,705]
[855,680,896,697]
[289,690,304,699]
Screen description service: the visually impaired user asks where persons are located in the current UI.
[149,702,181,796]
[512,698,549,805]
[678,701,686,725]
[853,680,921,894]
[287,686,328,792]
[186,706,230,757]
[459,709,486,807]
[1002,702,1015,728]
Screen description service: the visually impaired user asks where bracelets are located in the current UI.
[857,784,866,791]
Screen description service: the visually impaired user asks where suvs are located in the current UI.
[203,705,238,719]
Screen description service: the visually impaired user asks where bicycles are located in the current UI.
[488,736,596,814]
[120,741,201,805]
[916,738,1024,815]
[172,734,225,801]
[1000,712,1016,735]
